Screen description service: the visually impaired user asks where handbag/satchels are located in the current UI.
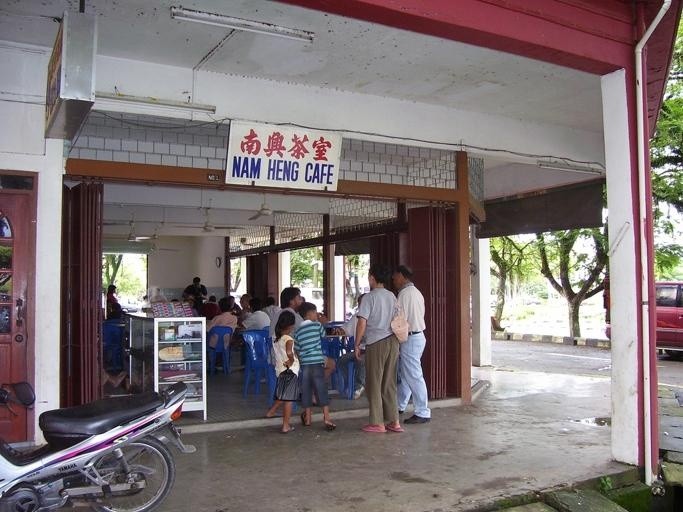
[388,300,411,342]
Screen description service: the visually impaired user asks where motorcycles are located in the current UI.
[0,381,195,512]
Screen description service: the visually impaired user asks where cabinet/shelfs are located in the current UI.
[125,311,210,422]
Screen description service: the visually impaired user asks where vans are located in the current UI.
[605,281,682,358]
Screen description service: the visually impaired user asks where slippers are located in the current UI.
[327,425,337,431]
[280,425,294,433]
[300,411,309,426]
[359,425,387,433]
[384,424,404,432]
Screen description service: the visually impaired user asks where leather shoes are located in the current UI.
[403,415,430,425]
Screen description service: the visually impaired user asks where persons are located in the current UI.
[207,296,237,350]
[203,296,219,318]
[262,298,280,322]
[392,265,432,424]
[267,311,300,435]
[185,298,198,317]
[333,293,367,399]
[241,300,270,331]
[269,287,337,408]
[354,266,405,433]
[106,285,120,316]
[293,301,337,427]
[182,277,208,310]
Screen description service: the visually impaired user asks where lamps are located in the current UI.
[171,6,317,45]
[95,91,217,117]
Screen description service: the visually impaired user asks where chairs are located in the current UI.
[322,320,369,401]
[104,320,128,376]
[239,330,270,400]
[205,326,233,378]
[262,327,271,332]
[265,338,303,413]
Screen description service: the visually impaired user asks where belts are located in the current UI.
[406,332,424,335]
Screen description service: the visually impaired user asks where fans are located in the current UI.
[146,207,178,226]
[138,228,176,241]
[246,193,290,222]
[129,243,181,257]
[105,201,145,210]
[111,213,153,228]
[176,218,246,234]
[177,197,243,218]
[109,229,152,244]
